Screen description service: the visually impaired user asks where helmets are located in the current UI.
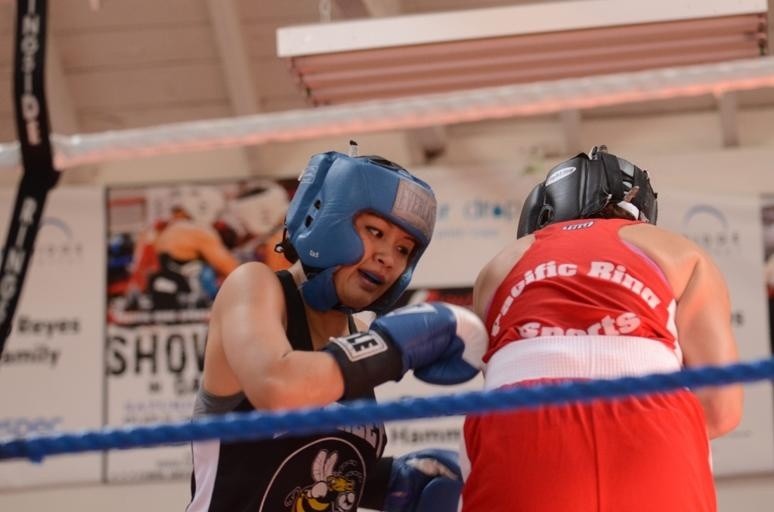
[219,177,290,247]
[283,150,437,315]
[516,144,658,240]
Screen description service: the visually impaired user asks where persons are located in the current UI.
[460,144,744,512]
[185,151,489,512]
[109,178,293,328]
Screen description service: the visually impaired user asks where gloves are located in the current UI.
[369,302,489,386]
[381,447,464,512]
